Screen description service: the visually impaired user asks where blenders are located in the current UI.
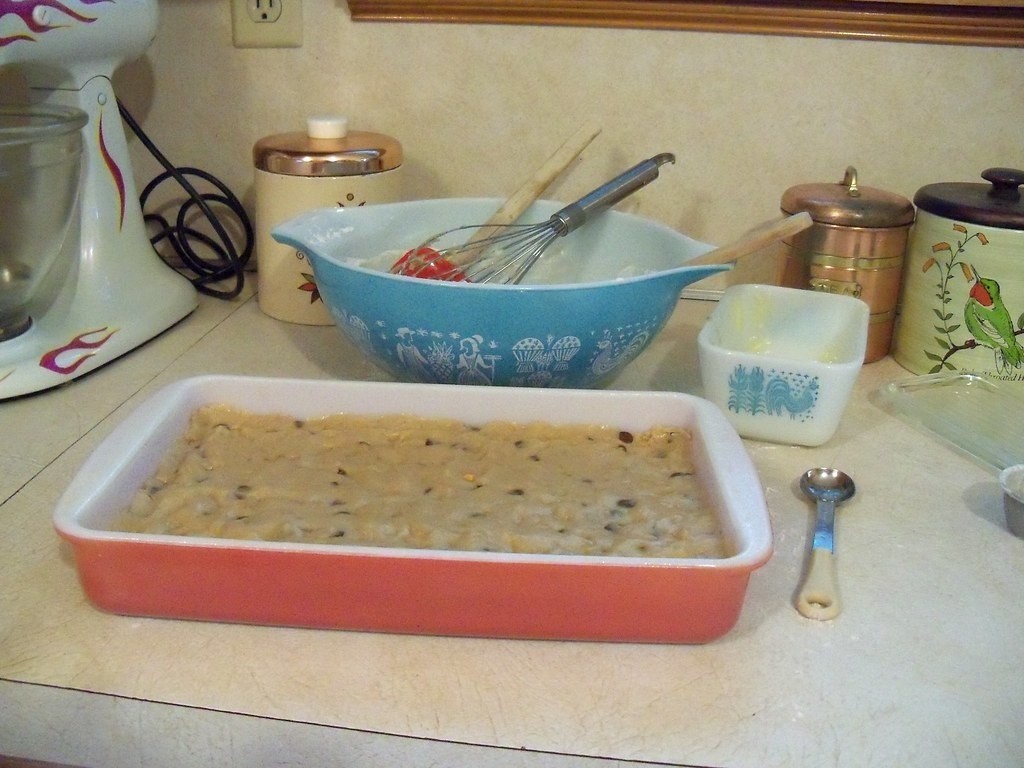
[0,1,201,401]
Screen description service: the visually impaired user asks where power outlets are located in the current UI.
[230,0,305,49]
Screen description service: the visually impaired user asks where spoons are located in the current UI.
[794,465,854,618]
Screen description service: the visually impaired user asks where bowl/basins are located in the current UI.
[272,197,740,386]
[53,374,779,644]
[695,281,868,446]
